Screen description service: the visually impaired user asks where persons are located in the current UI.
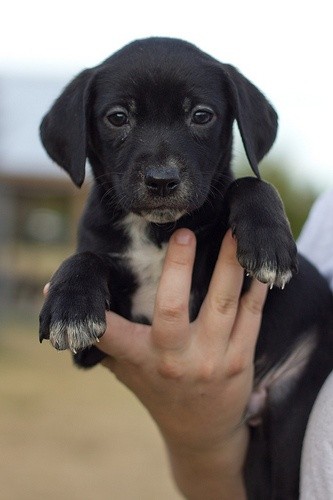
[42,188,332,500]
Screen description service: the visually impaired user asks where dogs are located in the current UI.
[36,38,333,500]
